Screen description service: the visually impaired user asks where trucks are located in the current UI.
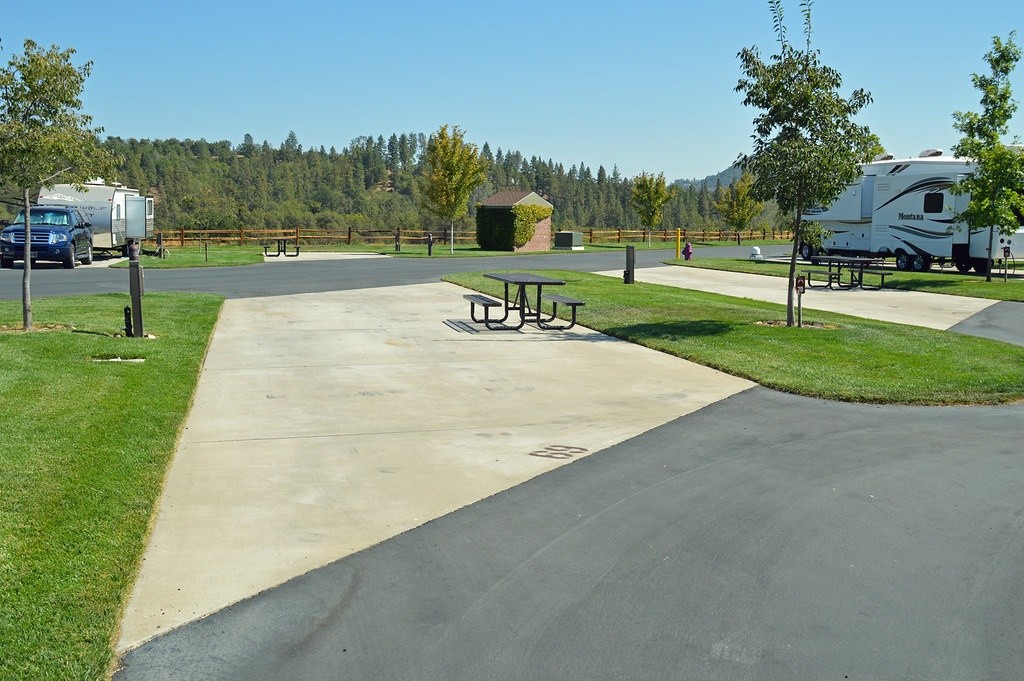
[795,144,1024,276]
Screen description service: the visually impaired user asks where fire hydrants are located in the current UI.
[683,241,693,261]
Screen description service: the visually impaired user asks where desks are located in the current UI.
[811,255,884,290]
[271,237,291,257]
[482,273,566,330]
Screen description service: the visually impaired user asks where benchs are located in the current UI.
[294,246,300,256]
[801,269,843,290]
[263,246,271,257]
[463,295,502,330]
[541,294,585,330]
[848,269,893,290]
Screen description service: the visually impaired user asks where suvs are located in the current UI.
[0,205,94,269]
